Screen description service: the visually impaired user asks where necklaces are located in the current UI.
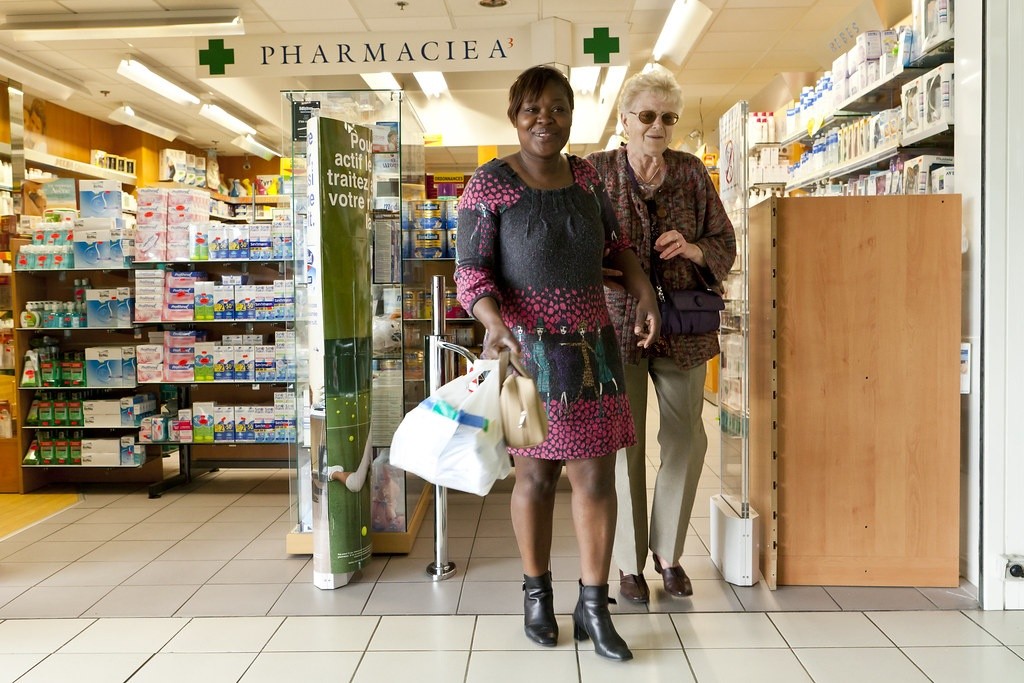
[628,153,664,187]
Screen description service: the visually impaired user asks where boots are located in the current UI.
[572,579,635,660]
[522,570,559,647]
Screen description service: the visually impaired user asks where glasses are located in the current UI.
[626,110,680,126]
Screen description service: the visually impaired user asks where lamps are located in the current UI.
[1,0,714,160]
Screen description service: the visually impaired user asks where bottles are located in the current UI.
[22,279,90,465]
[752,71,845,181]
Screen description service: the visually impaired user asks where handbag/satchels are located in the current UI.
[654,289,723,335]
[496,351,548,447]
[389,360,515,495]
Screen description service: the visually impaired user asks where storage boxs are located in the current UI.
[961,342,971,391]
[0,144,303,467]
[748,0,954,203]
[372,218,400,284]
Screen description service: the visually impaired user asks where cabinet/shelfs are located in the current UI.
[279,90,434,553]
[0,238,165,494]
[402,258,485,408]
[704,32,957,426]
[130,182,295,497]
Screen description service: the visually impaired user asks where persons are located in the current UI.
[453,67,662,662]
[582,64,737,604]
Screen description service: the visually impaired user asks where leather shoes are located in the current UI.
[653,553,693,597]
[619,569,650,602]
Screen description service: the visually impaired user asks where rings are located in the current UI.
[675,242,680,247]
